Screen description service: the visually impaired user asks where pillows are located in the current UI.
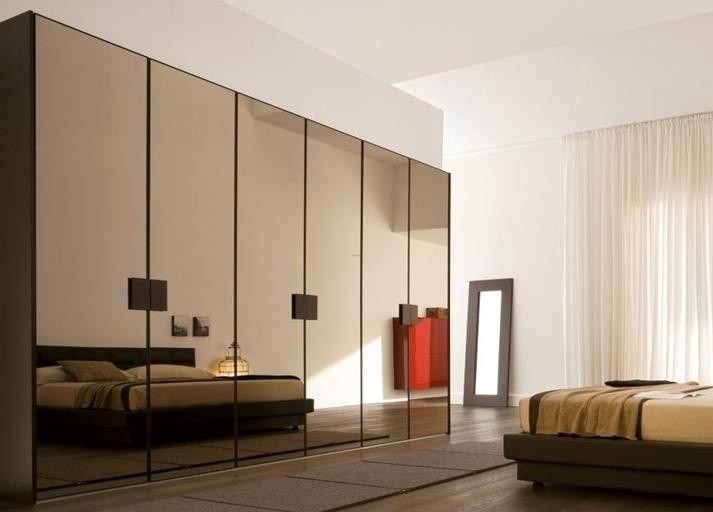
[38,359,217,383]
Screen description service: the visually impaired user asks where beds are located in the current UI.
[36,345,314,453]
[504,380,713,498]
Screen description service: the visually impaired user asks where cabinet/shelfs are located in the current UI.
[2,9,452,506]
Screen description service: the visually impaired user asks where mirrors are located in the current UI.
[463,278,513,407]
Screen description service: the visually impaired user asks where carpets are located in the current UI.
[106,441,519,512]
[36,430,390,491]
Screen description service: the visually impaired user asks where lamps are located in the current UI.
[219,341,248,377]
